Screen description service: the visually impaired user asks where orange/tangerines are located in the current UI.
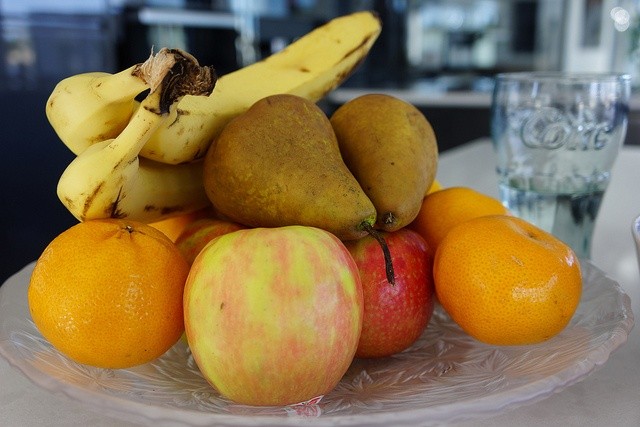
[29,217,191,369]
[432,215,582,345]
[416,186,508,253]
[425,180,442,196]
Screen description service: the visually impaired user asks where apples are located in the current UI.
[174,218,246,269]
[182,225,365,408]
[342,228,435,360]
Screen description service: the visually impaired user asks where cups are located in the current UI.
[489,69,631,262]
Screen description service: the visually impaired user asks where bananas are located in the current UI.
[44,10,382,223]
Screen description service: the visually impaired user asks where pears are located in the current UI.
[329,94,439,233]
[203,94,395,287]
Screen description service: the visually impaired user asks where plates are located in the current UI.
[1,257,636,426]
